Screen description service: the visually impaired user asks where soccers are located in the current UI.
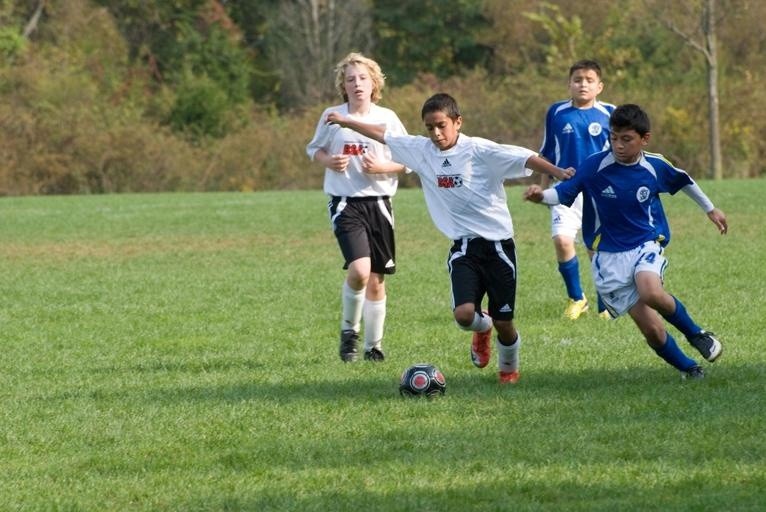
[400,363,447,400]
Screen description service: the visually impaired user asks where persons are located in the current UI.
[305,49,413,363]
[537,59,622,324]
[322,92,577,386]
[519,102,730,385]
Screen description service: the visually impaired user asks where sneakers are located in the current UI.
[689,328,723,362]
[563,294,588,319]
[340,328,360,363]
[687,365,704,379]
[599,310,611,321]
[500,371,519,382]
[471,310,492,368]
[365,347,383,362]
